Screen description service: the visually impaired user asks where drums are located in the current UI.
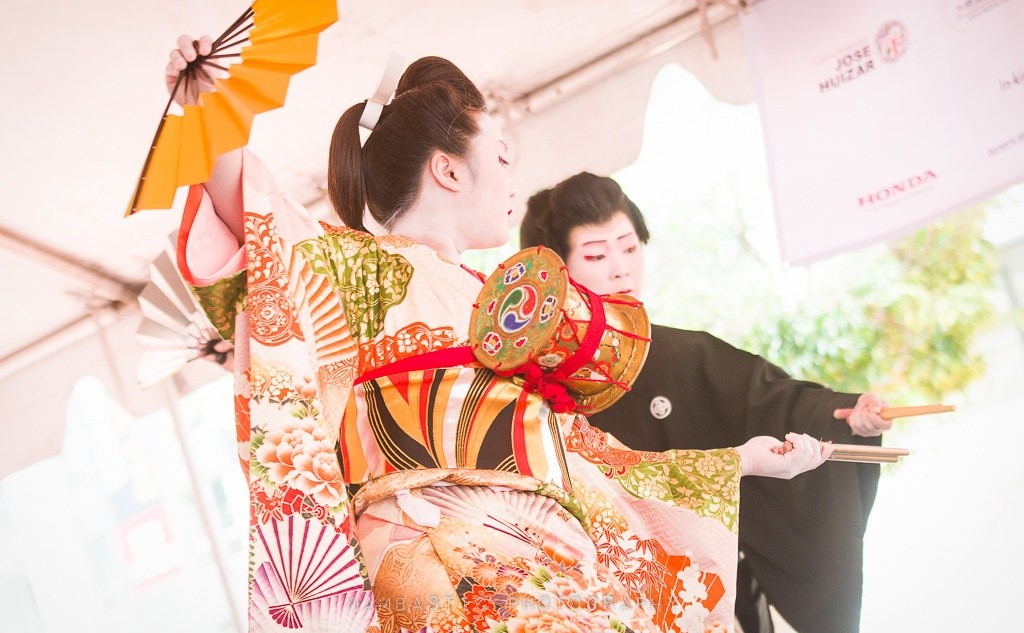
[469,245,652,415]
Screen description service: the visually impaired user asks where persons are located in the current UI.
[200,171,893,633]
[165,34,835,633]
